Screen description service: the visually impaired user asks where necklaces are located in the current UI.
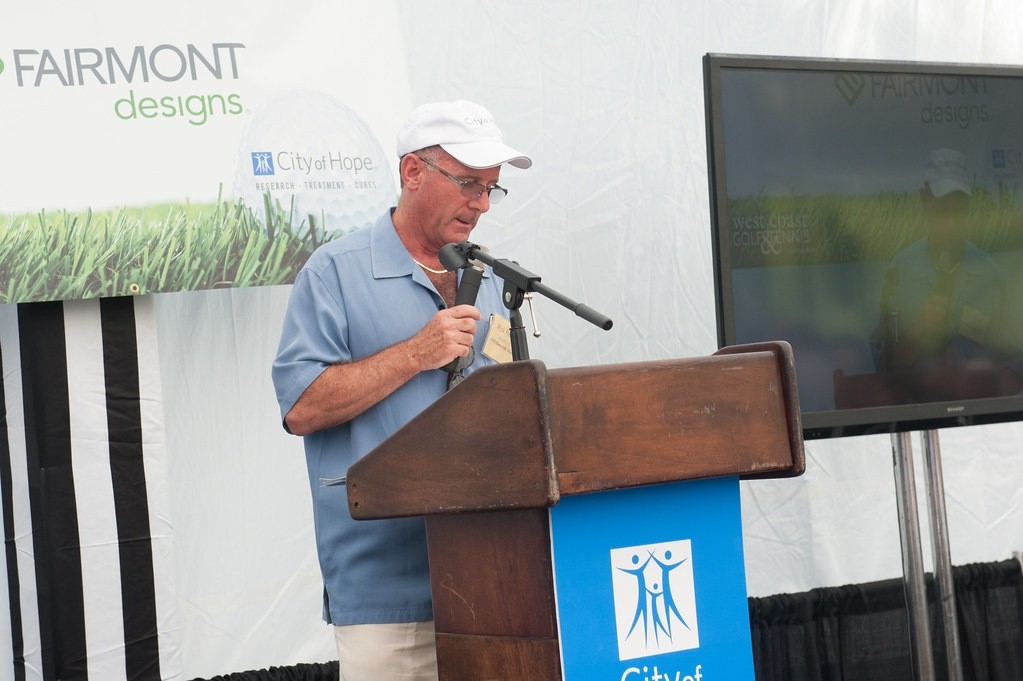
[414,259,448,274]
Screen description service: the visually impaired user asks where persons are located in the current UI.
[273,102,532,681]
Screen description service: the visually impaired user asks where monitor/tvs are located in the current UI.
[699,52,1023,443]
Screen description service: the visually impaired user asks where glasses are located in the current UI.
[449,344,475,389]
[416,155,508,204]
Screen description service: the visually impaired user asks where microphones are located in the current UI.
[440,264,484,375]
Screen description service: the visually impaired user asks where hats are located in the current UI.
[399,100,534,170]
